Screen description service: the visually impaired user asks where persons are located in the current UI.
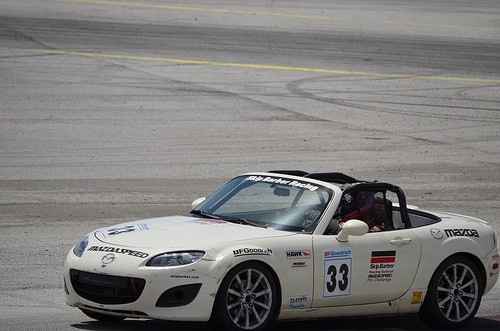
[305,181,385,234]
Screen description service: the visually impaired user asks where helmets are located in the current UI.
[339,182,375,222]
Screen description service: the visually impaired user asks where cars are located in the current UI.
[62,169,499,329]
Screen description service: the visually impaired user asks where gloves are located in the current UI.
[305,210,339,233]
